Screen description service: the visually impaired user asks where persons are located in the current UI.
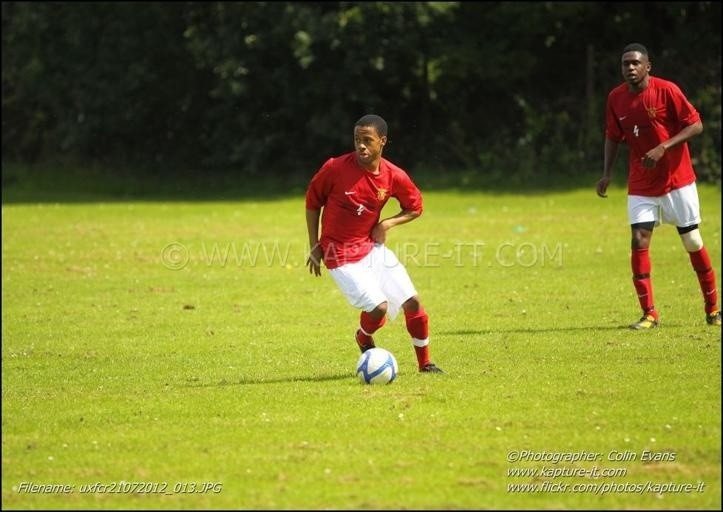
[596,43,722,331]
[305,113,445,375]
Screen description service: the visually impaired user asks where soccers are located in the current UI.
[357,348,397,384]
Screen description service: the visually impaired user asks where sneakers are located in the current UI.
[354,328,376,352]
[633,315,659,330]
[707,310,722,326]
[418,363,446,374]
